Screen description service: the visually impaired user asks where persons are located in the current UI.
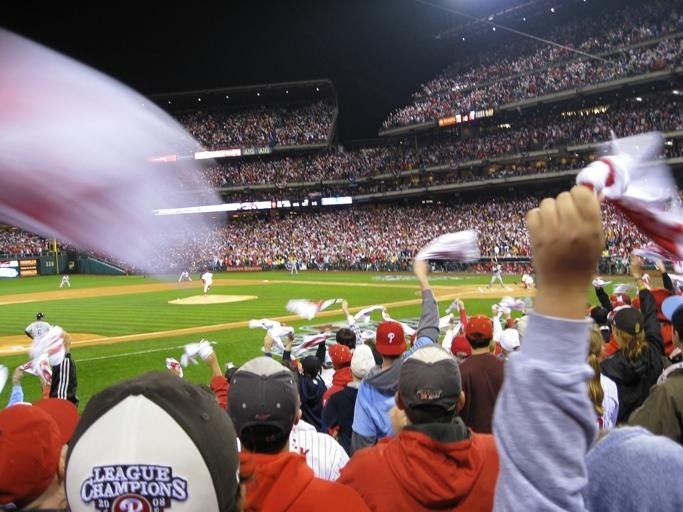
[0,224,141,273]
[459,300,468,332]
[8,367,24,407]
[223,208,396,275]
[60,273,70,288]
[174,102,334,151]
[440,262,482,274]
[169,168,210,191]
[423,230,462,245]
[265,323,285,357]
[325,344,376,458]
[649,289,674,356]
[203,351,230,413]
[549,137,683,172]
[587,323,618,444]
[322,344,353,441]
[225,368,239,383]
[491,297,530,358]
[228,357,369,511]
[470,96,683,159]
[380,1,683,130]
[587,277,630,344]
[597,255,663,427]
[397,203,482,229]
[458,314,508,435]
[318,345,335,389]
[396,229,424,272]
[236,360,350,484]
[441,317,472,364]
[316,331,326,366]
[283,331,328,432]
[39,375,52,398]
[49,331,80,411]
[491,297,527,319]
[491,185,682,512]
[337,343,501,512]
[218,182,365,205]
[201,269,213,296]
[345,259,439,457]
[367,154,547,194]
[652,262,675,356]
[178,270,192,282]
[150,221,223,270]
[25,313,52,340]
[481,199,543,291]
[629,296,682,446]
[599,194,683,264]
[210,133,470,186]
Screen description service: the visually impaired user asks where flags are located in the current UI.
[19,339,53,381]
[354,306,386,323]
[165,357,183,378]
[439,298,461,330]
[294,332,329,355]
[286,298,345,320]
[414,230,480,262]
[272,326,294,350]
[382,310,416,336]
[226,362,234,370]
[250,318,280,330]
[45,326,65,366]
[183,339,214,367]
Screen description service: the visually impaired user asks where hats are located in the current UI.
[516,315,528,337]
[610,293,632,305]
[328,344,353,367]
[350,345,376,378]
[299,356,322,374]
[451,336,472,359]
[466,314,493,340]
[632,289,671,308]
[398,343,462,418]
[606,304,645,335]
[661,295,683,334]
[323,350,333,368]
[227,357,299,450]
[376,321,407,355]
[37,312,44,318]
[497,328,520,353]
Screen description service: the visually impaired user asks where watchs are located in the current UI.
[635,276,642,281]
